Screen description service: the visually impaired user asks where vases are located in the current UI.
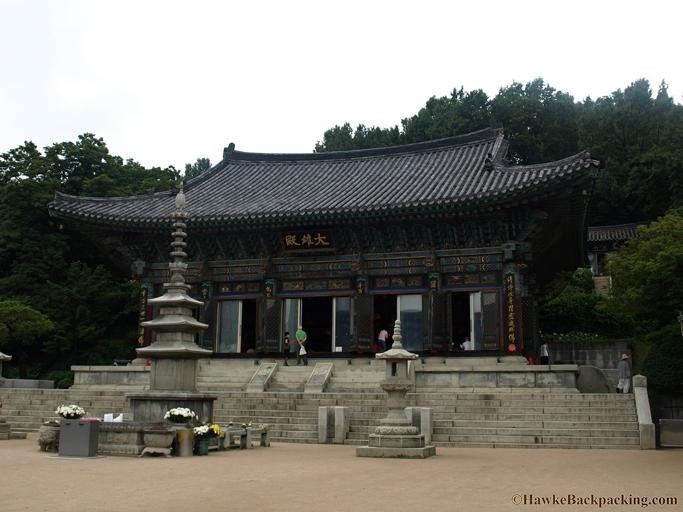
[196,440,208,455]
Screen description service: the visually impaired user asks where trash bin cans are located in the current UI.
[177,429,194,457]
[58,419,99,456]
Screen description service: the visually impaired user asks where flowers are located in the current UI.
[54,403,86,419]
[163,405,199,423]
[193,422,224,441]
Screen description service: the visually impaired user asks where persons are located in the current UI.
[460,336,471,350]
[295,326,308,366]
[540,342,550,365]
[283,332,290,366]
[378,328,390,352]
[616,354,631,393]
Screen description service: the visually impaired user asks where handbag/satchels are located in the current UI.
[300,346,307,355]
